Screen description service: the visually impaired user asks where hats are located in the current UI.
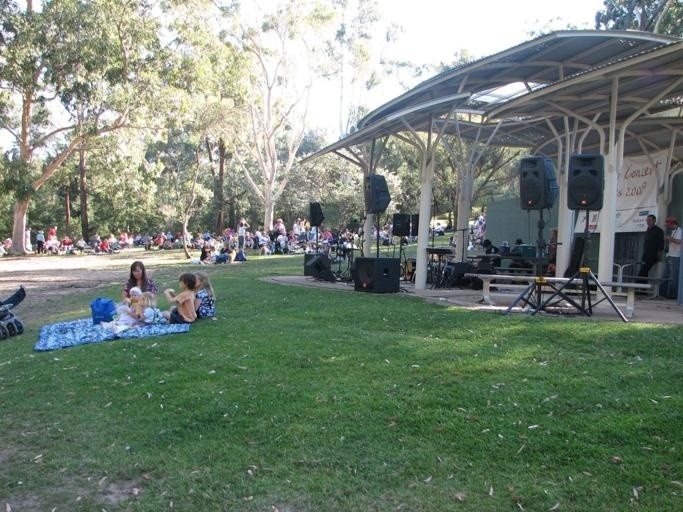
[663,216,675,227]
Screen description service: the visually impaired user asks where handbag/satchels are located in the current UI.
[90,297,117,325]
[0,312,23,340]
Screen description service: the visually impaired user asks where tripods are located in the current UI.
[429,253,451,290]
[531,210,628,323]
[503,210,592,318]
[340,222,354,277]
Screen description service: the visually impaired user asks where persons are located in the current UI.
[159,272,197,325]
[477,239,499,291]
[383,223,444,244]
[635,214,663,296]
[658,215,682,299]
[193,272,216,320]
[546,227,556,278]
[467,204,487,251]
[98,261,158,336]
[0,225,184,255]
[190,217,360,265]
[507,237,533,285]
[124,285,151,323]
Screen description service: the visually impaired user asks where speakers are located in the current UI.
[304,254,328,276]
[392,214,410,237]
[364,175,390,214]
[411,214,419,237]
[567,154,605,210]
[354,257,401,293]
[310,202,324,226]
[520,155,559,210]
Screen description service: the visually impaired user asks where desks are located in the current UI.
[471,254,546,274]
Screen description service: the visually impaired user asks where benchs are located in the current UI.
[462,270,653,320]
